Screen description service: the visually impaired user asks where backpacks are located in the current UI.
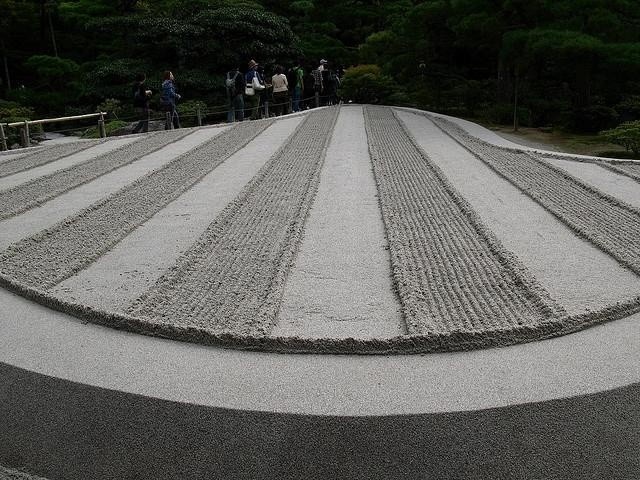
[287,67,299,88]
[225,71,238,96]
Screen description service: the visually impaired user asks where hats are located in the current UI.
[320,59,328,64]
[248,60,259,69]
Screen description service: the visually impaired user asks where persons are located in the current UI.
[132,72,152,133]
[225,58,341,120]
[161,70,181,130]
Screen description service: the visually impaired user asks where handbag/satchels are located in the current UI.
[245,84,256,95]
[252,72,265,90]
